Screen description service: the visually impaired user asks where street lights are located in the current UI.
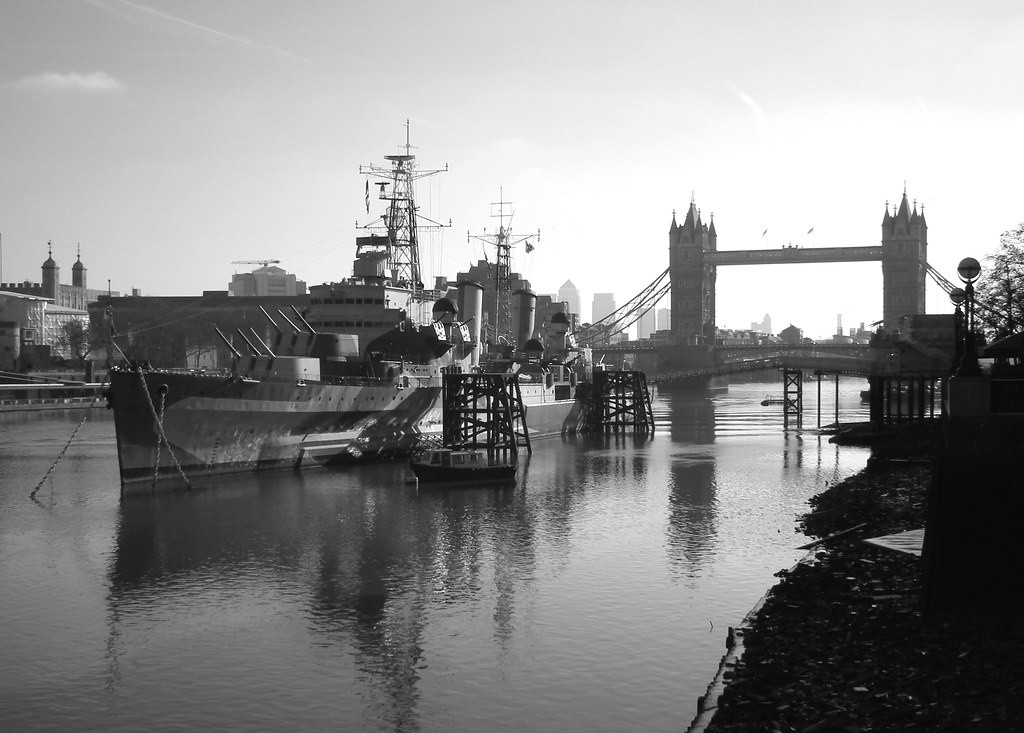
[949,288,966,376]
[953,258,989,379]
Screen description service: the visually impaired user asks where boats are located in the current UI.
[89,117,661,484]
